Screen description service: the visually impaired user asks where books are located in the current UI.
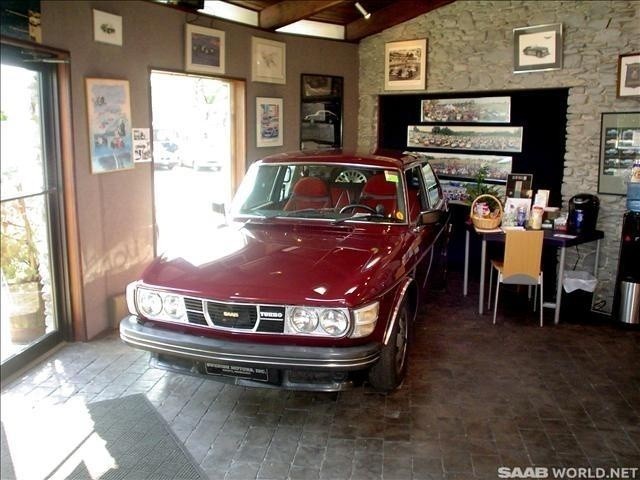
[504,174,560,224]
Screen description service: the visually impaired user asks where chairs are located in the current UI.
[283,176,333,213]
[354,174,398,221]
[487,228,544,328]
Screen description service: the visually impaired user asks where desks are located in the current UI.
[460,219,604,326]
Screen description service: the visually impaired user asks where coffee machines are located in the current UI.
[568,192,600,235]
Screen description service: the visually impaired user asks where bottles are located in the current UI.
[517,206,528,227]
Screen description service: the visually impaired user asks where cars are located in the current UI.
[180,148,223,172]
[261,117,279,136]
[118,153,452,394]
[390,64,418,79]
[522,46,550,58]
[604,128,640,177]
[410,132,520,149]
[423,102,506,120]
[426,159,509,179]
[304,110,335,124]
[441,185,506,204]
[153,141,180,171]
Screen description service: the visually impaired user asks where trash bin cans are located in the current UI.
[619,277,640,324]
[559,270,598,324]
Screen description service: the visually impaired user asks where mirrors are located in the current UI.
[300,73,344,152]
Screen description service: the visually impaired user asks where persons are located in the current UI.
[631,67,638,80]
[120,120,125,137]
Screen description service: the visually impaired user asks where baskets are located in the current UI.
[471,194,503,229]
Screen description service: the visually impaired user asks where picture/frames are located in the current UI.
[512,22,563,74]
[597,111,640,195]
[407,96,523,207]
[85,76,134,175]
[256,96,283,148]
[184,22,226,76]
[251,35,286,85]
[617,52,640,98]
[384,38,426,91]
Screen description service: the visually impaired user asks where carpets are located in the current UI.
[0,393,210,480]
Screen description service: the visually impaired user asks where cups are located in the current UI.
[530,206,544,230]
